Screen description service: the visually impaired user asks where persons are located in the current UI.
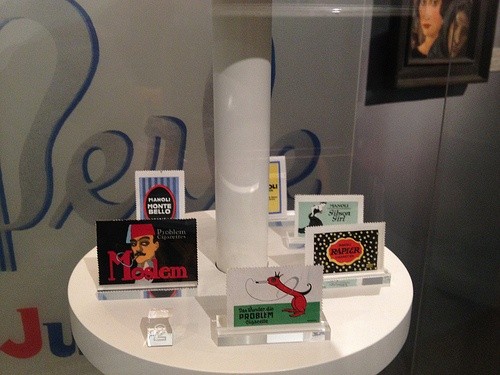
[428,1,472,61]
[409,0,451,58]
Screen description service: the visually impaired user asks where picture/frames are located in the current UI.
[392,1,500,87]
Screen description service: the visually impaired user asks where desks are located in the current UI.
[66,208,416,375]
